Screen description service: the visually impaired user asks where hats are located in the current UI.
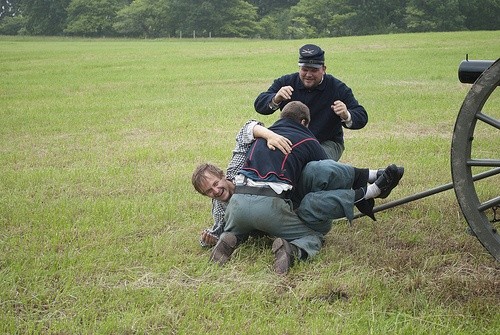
[298,43,325,69]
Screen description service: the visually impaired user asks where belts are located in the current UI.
[232,186,286,198]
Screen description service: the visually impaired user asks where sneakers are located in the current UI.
[369,165,404,199]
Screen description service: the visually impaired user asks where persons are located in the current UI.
[192,117,404,252]
[254,44,368,162]
[210,100,329,275]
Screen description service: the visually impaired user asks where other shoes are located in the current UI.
[207,232,237,268]
[271,238,298,277]
